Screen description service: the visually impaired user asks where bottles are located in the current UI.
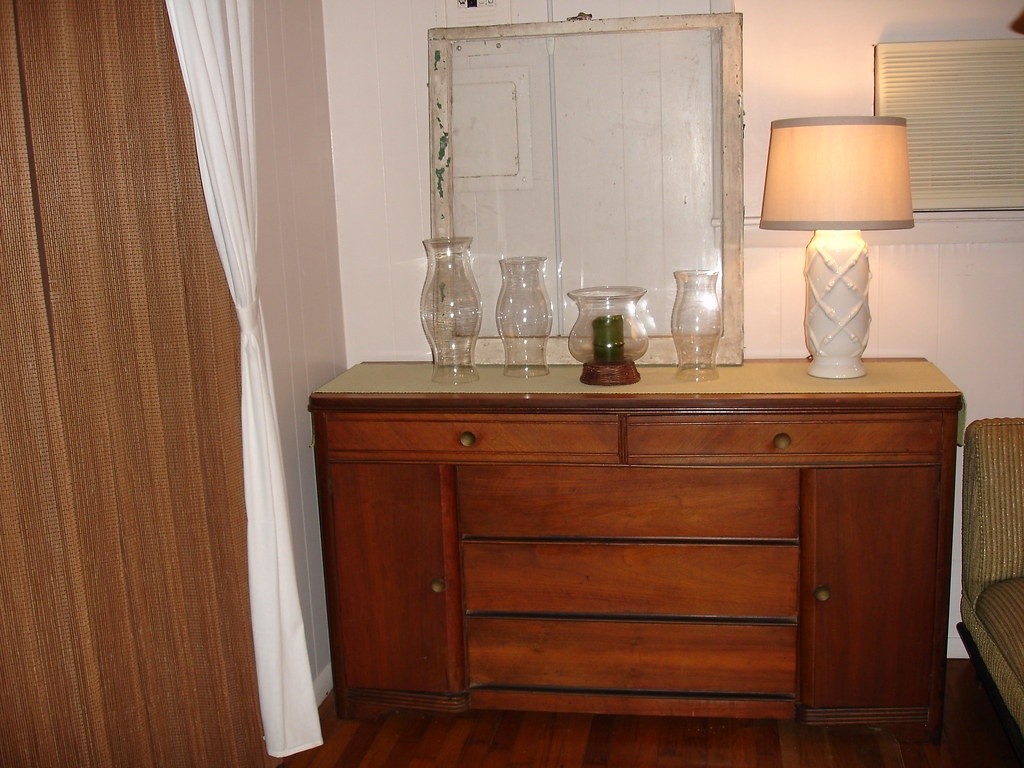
[418,237,481,385]
[496,257,553,378]
[669,270,721,386]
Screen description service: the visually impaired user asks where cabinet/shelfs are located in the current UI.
[306,360,963,745]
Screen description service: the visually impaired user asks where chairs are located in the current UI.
[956,419,1024,767]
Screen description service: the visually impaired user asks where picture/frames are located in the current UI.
[428,12,744,366]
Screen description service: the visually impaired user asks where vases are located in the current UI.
[669,270,725,383]
[497,256,552,379]
[561,285,647,386]
[421,236,482,384]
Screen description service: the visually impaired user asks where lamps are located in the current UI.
[760,116,914,380]
